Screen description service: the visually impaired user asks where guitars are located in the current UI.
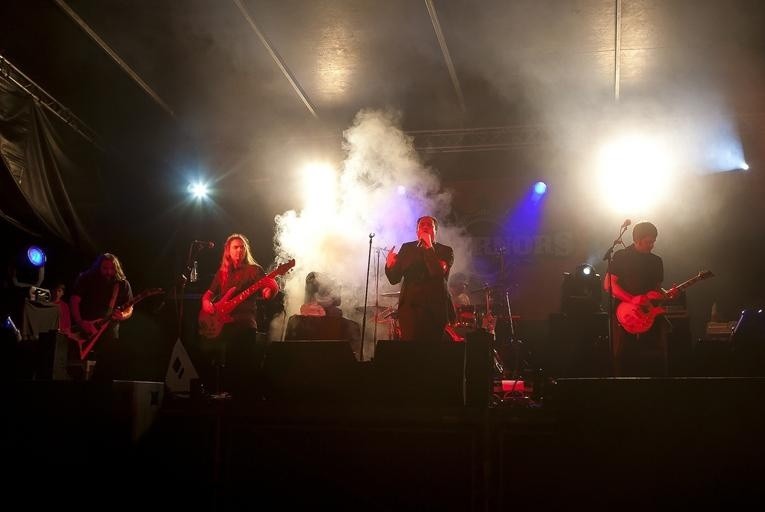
[198,260,295,337]
[615,270,714,334]
[67,288,166,361]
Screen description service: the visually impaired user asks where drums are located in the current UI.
[449,304,481,328]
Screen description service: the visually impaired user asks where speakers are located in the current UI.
[230,339,356,400]
[372,338,495,404]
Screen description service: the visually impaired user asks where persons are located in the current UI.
[385,215,455,343]
[447,273,470,310]
[50,284,70,333]
[199,234,277,350]
[69,254,135,362]
[604,222,681,355]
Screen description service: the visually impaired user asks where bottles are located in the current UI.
[190,261,197,282]
[710,302,719,321]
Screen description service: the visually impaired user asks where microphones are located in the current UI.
[415,230,427,251]
[192,239,217,250]
[609,237,623,251]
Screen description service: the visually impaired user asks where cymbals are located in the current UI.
[355,306,388,312]
[381,291,400,297]
